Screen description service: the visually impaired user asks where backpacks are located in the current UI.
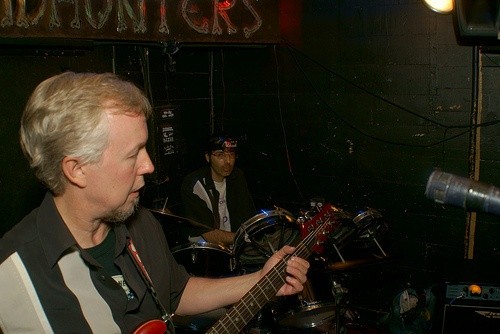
[376,288,431,334]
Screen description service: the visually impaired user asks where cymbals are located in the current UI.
[149,208,192,222]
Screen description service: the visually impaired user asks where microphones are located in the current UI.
[424,171,500,214]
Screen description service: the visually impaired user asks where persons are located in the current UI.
[0,71,310,334]
[184,132,262,247]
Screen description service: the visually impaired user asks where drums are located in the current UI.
[279,301,346,334]
[233,206,301,267]
[169,239,241,280]
[353,207,383,239]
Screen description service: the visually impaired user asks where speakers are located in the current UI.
[452,0,500,46]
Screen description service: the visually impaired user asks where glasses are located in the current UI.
[210,150,237,160]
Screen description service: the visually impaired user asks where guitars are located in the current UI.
[131,199,346,334]
